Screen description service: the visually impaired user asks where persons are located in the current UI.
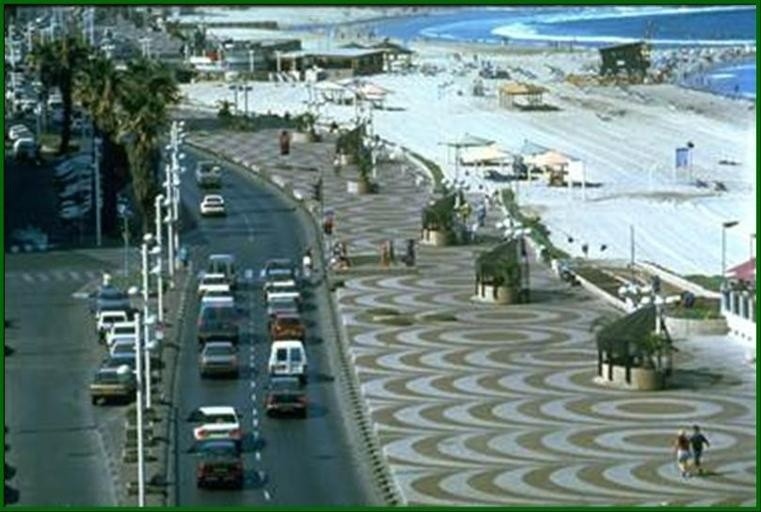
[276,108,419,288]
[672,429,695,479]
[449,178,578,285]
[686,424,711,476]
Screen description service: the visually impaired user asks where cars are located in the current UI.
[262,376,308,418]
[89,284,138,406]
[195,253,239,343]
[197,339,241,379]
[194,438,244,489]
[194,160,223,188]
[260,257,306,343]
[199,193,227,216]
[192,405,243,442]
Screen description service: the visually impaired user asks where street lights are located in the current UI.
[720,220,738,283]
[133,119,191,508]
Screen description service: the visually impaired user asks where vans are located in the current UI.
[268,339,309,378]
